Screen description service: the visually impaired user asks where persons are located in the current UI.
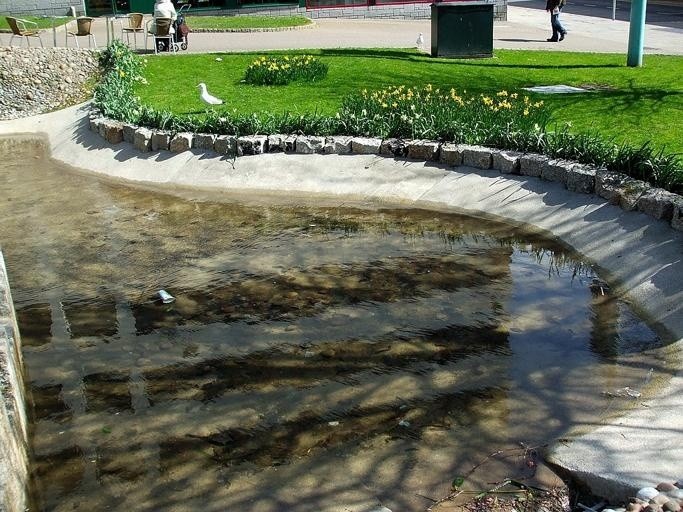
[545,1,567,42]
[149,1,178,36]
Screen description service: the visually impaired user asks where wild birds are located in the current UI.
[416,32,424,49]
[195,82,226,112]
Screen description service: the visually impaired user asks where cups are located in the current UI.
[159,290,176,304]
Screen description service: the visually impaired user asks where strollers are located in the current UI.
[157,4,192,52]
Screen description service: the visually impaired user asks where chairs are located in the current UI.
[5,12,175,54]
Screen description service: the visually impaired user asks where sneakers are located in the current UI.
[547,37,557,42]
[559,29,566,41]
